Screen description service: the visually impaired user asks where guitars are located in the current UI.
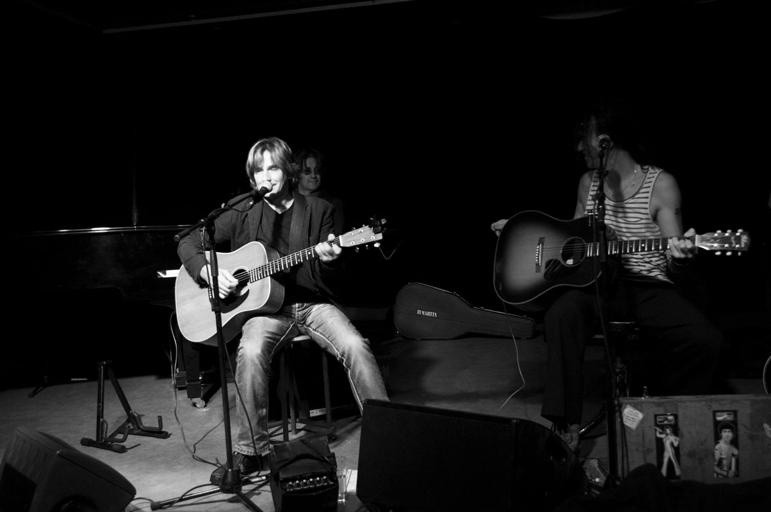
[174,217,382,348]
[493,212,752,309]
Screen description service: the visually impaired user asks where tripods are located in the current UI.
[589,242,659,512]
[151,228,273,512]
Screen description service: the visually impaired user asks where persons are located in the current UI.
[176,138,391,485]
[490,103,724,456]
[655,425,681,478]
[291,151,344,236]
[714,421,739,479]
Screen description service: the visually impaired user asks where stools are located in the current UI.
[262,330,340,449]
[573,318,656,448]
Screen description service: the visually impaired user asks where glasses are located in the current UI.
[301,166,321,176]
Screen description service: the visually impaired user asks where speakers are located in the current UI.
[614,394,771,498]
[356,398,589,512]
[0,425,136,512]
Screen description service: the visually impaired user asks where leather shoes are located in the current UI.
[211,450,264,486]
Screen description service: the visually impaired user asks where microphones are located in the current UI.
[597,133,611,150]
[253,181,273,203]
